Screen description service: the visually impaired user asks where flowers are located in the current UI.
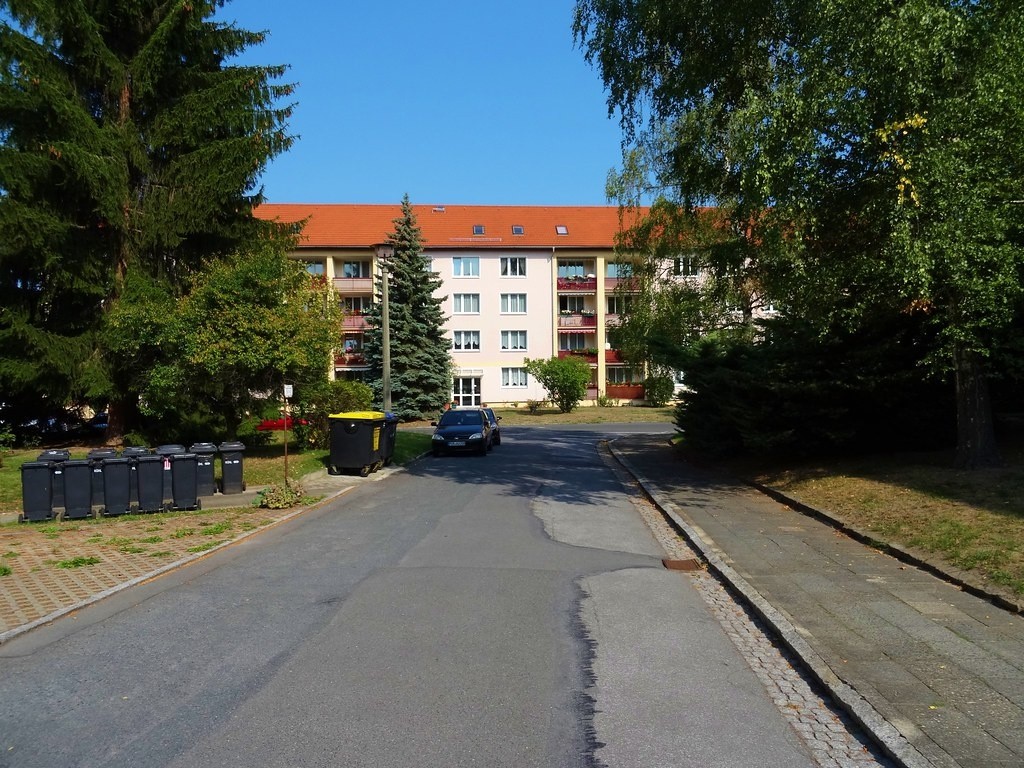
[560,310,572,317]
[580,309,595,315]
[345,346,353,354]
[360,308,368,315]
[354,309,360,316]
[566,276,575,282]
[577,275,587,282]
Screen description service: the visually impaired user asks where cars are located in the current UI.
[431,409,493,456]
[483,408,502,445]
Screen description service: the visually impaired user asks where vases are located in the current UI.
[584,314,594,317]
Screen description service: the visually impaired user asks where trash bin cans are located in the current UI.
[98,457,135,518]
[167,453,202,511]
[156,444,186,501]
[37,448,72,507]
[325,410,398,475]
[18,460,58,525]
[220,442,247,495]
[57,460,97,523]
[85,447,117,505]
[133,454,168,515]
[190,442,218,497]
[120,446,151,503]
